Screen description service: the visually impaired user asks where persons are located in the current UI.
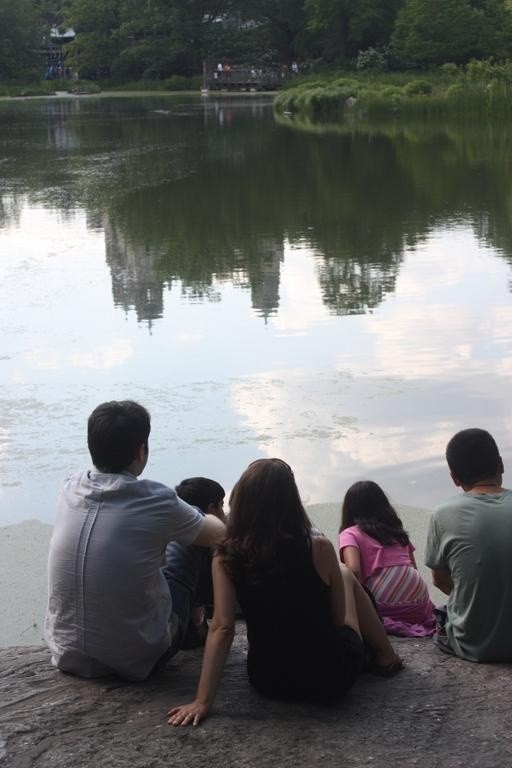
[163,476,230,651]
[165,458,404,728]
[425,427,512,664]
[44,400,230,685]
[335,481,441,638]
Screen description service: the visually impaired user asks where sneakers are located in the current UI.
[185,617,208,648]
